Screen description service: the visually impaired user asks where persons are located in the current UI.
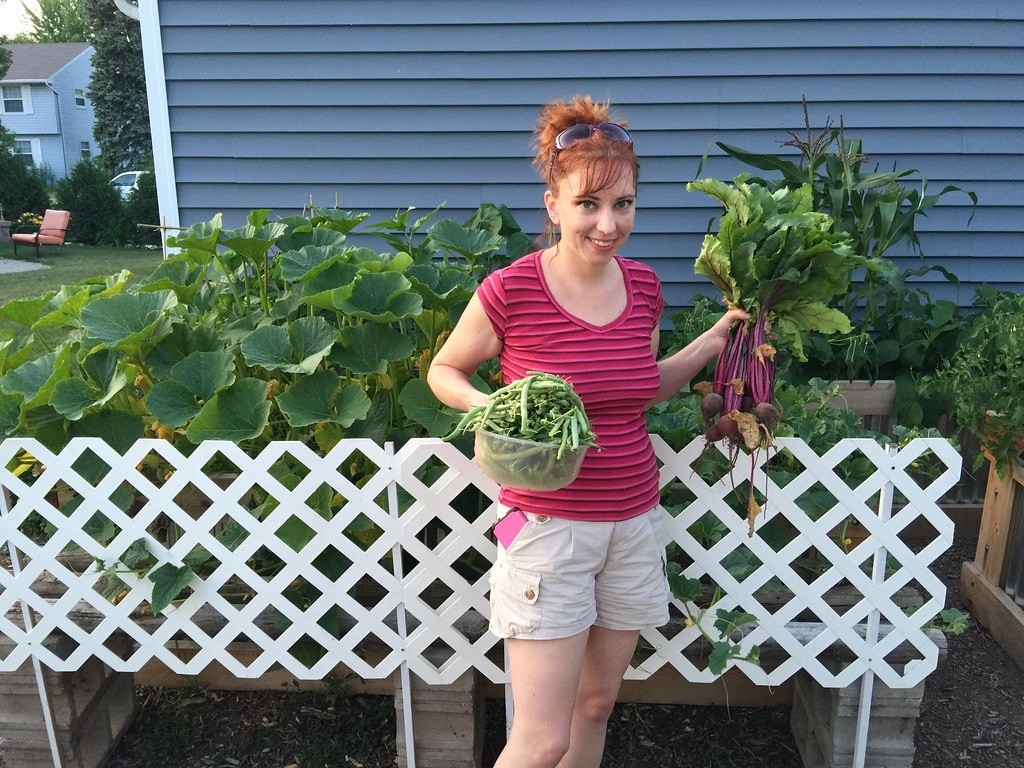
[427,94,772,768]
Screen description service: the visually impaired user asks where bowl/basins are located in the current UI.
[474,429,590,492]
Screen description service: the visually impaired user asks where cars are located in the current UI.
[109,168,151,202]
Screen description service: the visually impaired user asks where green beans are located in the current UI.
[441,371,603,489]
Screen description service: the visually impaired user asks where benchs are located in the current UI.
[11,208,70,258]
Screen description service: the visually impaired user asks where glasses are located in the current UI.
[548,122,634,190]
